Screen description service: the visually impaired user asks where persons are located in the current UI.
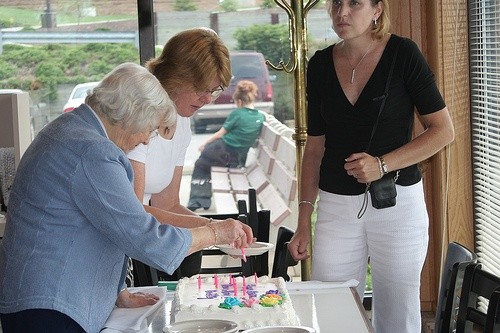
[124,28,257,249]
[0,61,257,333]
[287,0,455,333]
[187,80,266,211]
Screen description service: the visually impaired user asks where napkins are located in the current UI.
[104,286,167,333]
[286,279,360,290]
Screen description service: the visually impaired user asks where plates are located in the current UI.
[241,325,315,333]
[214,241,276,255]
[162,318,239,333]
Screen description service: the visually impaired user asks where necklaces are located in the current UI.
[344,39,375,83]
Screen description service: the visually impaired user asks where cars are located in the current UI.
[63,81,101,113]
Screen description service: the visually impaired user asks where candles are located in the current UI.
[241,248,247,262]
[197,273,257,296]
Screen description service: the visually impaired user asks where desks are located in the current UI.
[101,278,375,333]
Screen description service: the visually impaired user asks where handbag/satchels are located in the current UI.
[370,174,398,210]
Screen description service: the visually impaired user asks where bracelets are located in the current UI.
[299,200,315,208]
[205,224,218,250]
[374,156,389,178]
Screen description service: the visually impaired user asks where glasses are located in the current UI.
[191,83,225,98]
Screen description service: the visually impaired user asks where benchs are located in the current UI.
[211,109,297,227]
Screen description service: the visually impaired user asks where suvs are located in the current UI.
[189,52,277,134]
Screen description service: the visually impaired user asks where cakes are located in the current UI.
[170,276,300,330]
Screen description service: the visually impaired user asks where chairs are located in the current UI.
[132,189,298,287]
[434,241,500,333]
